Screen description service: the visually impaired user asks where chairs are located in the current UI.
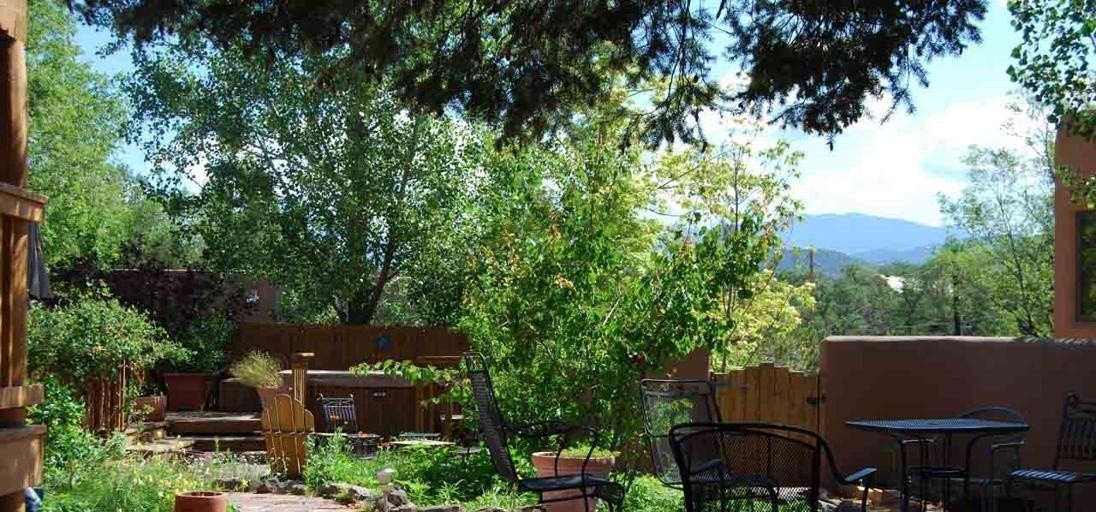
[901,391,1095,511]
[460,349,626,511]
[316,394,384,457]
[638,376,880,512]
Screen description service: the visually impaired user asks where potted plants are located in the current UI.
[129,338,292,421]
[528,444,622,512]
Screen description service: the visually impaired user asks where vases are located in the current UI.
[175,490,226,512]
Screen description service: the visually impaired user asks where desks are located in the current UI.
[843,415,1031,511]
[388,440,457,459]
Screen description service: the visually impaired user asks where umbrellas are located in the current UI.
[29,219,53,300]
